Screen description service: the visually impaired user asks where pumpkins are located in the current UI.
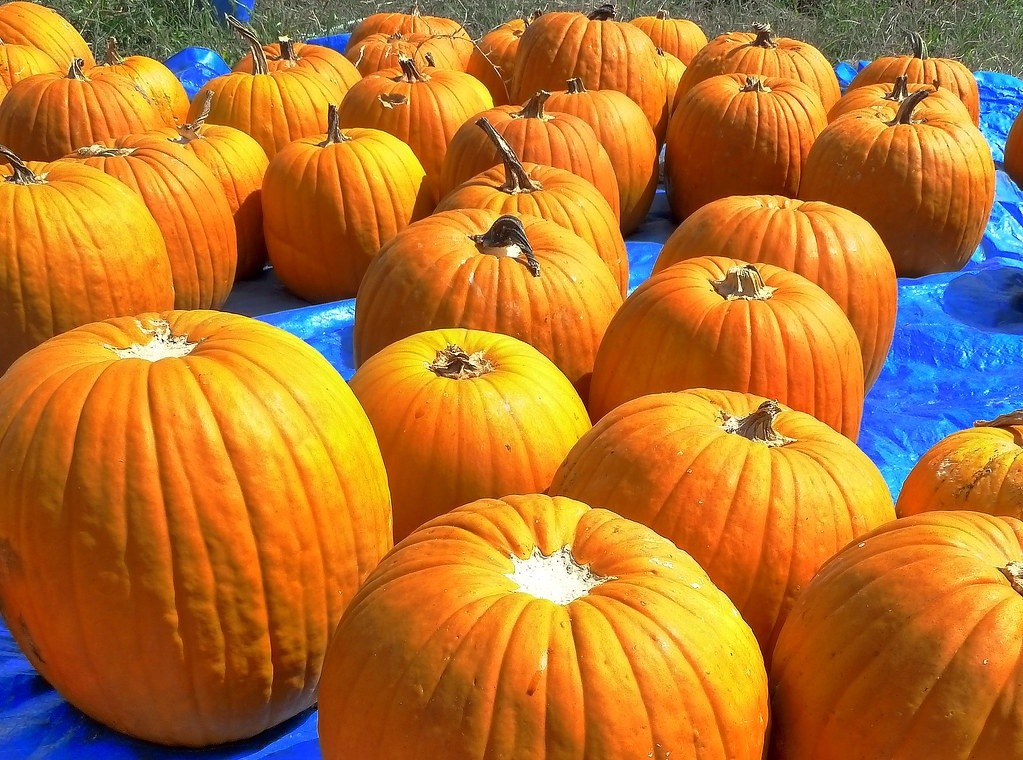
[0,1,1023,760]
